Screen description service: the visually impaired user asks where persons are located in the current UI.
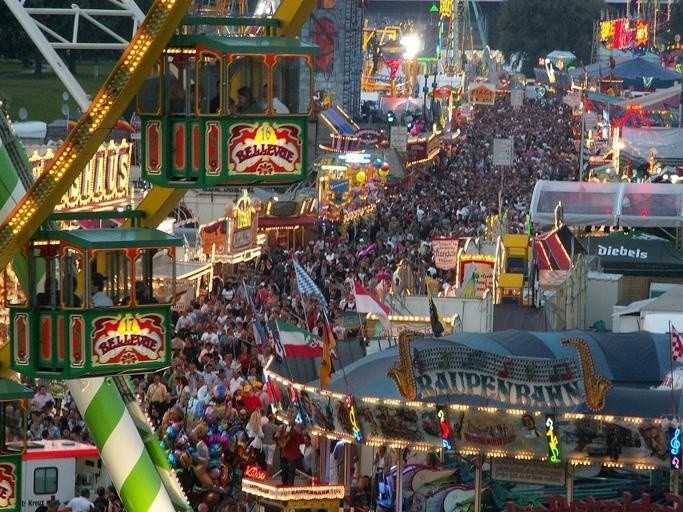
[0,372,95,446]
[32,483,123,511]
[164,81,291,113]
[637,425,668,459]
[120,237,486,510]
[300,70,589,237]
[34,272,162,309]
[518,412,542,439]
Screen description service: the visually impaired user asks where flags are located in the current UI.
[670,324,682,362]
